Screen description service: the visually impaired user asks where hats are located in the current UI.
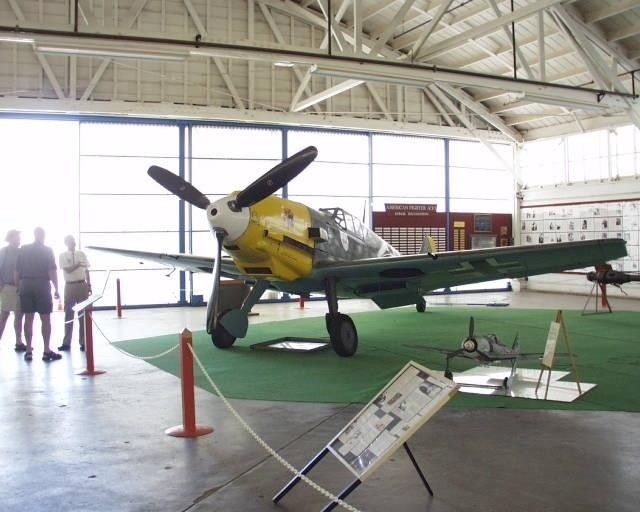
[5,230,20,242]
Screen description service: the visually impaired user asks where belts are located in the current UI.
[66,280,85,284]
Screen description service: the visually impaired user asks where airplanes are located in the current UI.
[85,146,628,357]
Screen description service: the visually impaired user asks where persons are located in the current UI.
[0,228,62,360]
[58,235,92,351]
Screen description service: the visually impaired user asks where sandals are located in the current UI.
[42,351,61,360]
[15,344,33,360]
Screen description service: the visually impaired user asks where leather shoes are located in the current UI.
[58,345,70,349]
[80,346,84,350]
